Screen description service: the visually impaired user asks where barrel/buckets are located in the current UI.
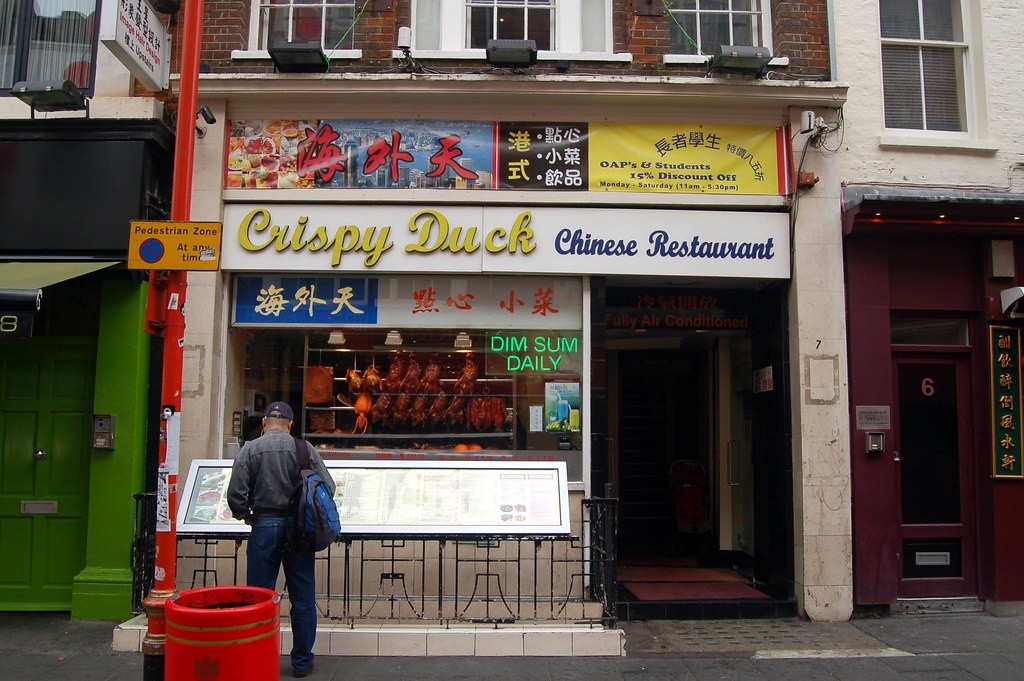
[162,586,280,681]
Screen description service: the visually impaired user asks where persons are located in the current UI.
[226,401,336,679]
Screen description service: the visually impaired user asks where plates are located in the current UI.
[228,120,302,188]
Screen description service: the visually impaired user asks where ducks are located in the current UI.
[346,351,508,434]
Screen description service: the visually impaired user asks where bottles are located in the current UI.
[548,387,558,421]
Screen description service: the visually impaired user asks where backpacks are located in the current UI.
[284,437,341,552]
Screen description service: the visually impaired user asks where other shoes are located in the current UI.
[293,665,313,678]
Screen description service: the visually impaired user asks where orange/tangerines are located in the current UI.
[455,444,481,452]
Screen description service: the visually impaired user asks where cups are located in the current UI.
[558,400,568,421]
[570,409,580,432]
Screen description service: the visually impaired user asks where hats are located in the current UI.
[265,402,293,419]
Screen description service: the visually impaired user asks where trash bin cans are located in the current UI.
[165,585,281,681]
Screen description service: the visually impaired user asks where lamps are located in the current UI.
[327,329,346,344]
[486,39,537,74]
[267,41,329,73]
[710,44,774,79]
[454,332,472,348]
[384,330,403,345]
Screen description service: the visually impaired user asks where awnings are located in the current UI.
[0,259,122,339]
